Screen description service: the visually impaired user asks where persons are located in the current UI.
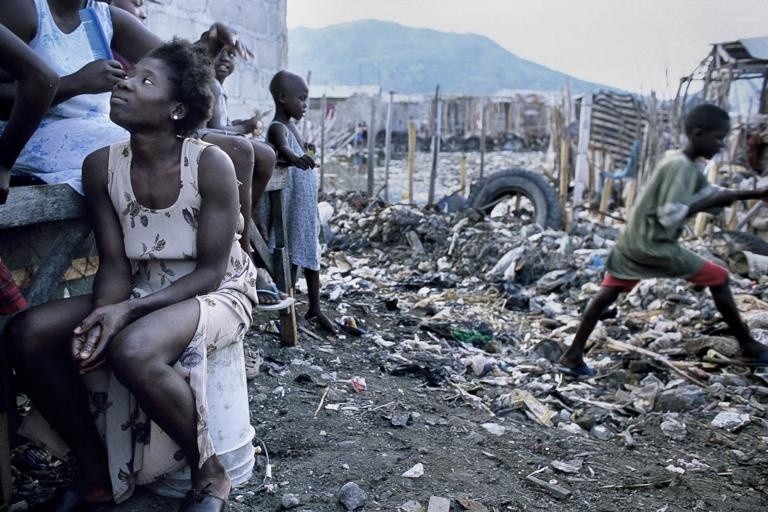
[560,105,768,376]
[255,70,337,335]
[0,0,276,511]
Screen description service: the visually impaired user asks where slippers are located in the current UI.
[554,364,597,377]
[178,482,232,512]
[334,315,367,334]
[736,346,768,363]
[258,320,281,334]
[73,469,112,503]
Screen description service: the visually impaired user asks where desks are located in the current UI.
[0,166,297,347]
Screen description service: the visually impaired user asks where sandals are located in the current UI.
[244,344,264,379]
[256,268,282,304]
